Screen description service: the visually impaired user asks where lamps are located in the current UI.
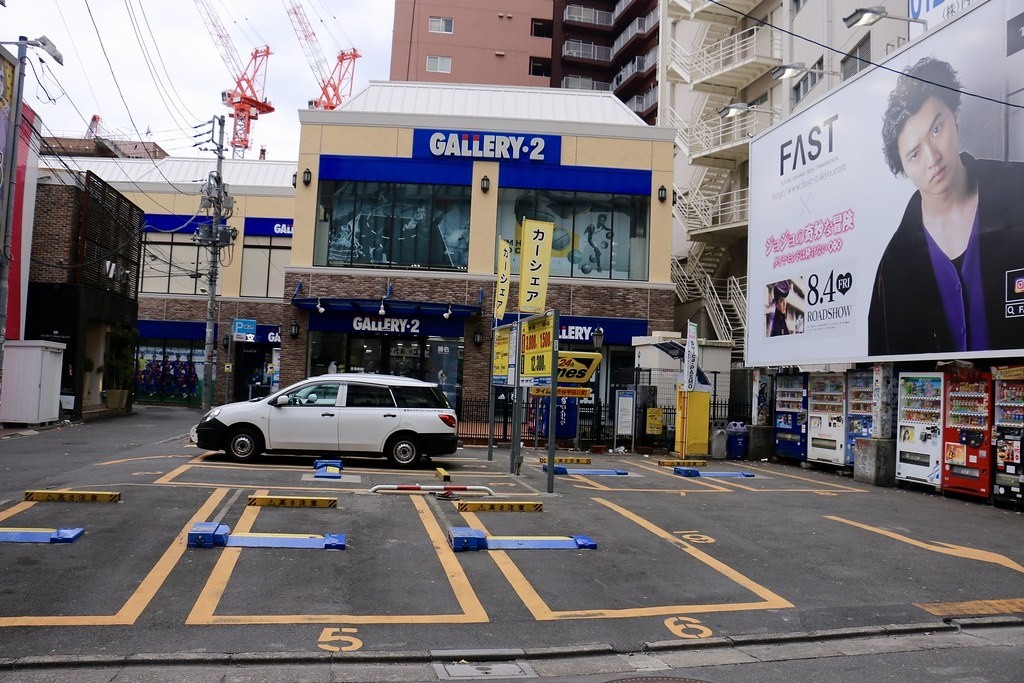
[317,297,325,313]
[771,62,845,81]
[481,175,489,193]
[303,168,311,187]
[292,172,297,188]
[716,103,782,120]
[658,184,666,203]
[289,319,299,337]
[474,328,483,346]
[843,8,927,32]
[443,303,453,319]
[673,189,677,206]
[378,297,385,315]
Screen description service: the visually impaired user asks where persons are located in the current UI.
[868,58,1024,356]
[403,202,427,231]
[770,280,799,337]
[579,214,614,273]
[368,213,400,264]
[136,352,199,398]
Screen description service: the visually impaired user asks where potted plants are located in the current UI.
[82,356,95,400]
[104,325,140,409]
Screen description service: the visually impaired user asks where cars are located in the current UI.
[195,372,459,470]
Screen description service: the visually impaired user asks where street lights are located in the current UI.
[200,288,216,411]
[590,325,607,445]
[0,34,65,393]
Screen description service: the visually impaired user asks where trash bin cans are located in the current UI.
[727,422,748,460]
[709,421,727,460]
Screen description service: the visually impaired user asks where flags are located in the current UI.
[494,239,511,320]
[519,219,554,313]
